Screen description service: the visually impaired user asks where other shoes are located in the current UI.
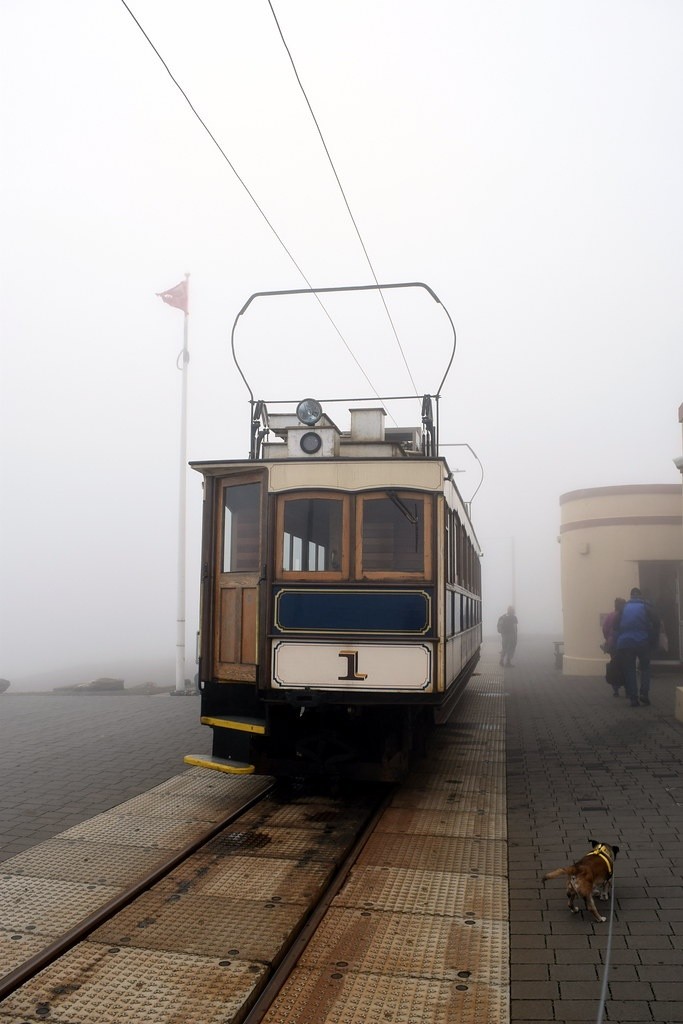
[506,661,513,667]
[612,688,619,697]
[499,660,504,667]
[631,697,640,708]
[641,693,651,704]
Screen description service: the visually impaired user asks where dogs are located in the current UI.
[542,838,619,922]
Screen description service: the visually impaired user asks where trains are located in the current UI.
[184,282,484,800]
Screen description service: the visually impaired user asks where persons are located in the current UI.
[600,596,635,699]
[497,606,519,669]
[614,588,660,708]
